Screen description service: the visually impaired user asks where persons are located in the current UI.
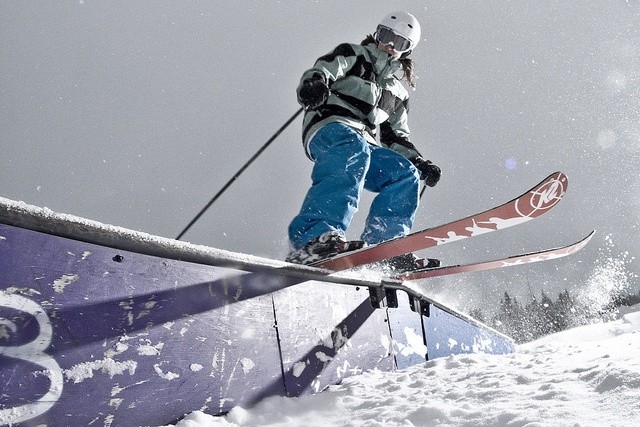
[285,10,441,271]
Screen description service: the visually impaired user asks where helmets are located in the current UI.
[376,10,421,51]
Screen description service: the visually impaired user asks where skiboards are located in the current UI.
[305,172,596,281]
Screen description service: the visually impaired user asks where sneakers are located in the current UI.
[285,229,347,265]
[381,252,442,273]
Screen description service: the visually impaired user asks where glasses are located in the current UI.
[376,25,413,54]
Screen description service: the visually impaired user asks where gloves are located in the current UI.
[416,162,443,187]
[297,72,329,112]
